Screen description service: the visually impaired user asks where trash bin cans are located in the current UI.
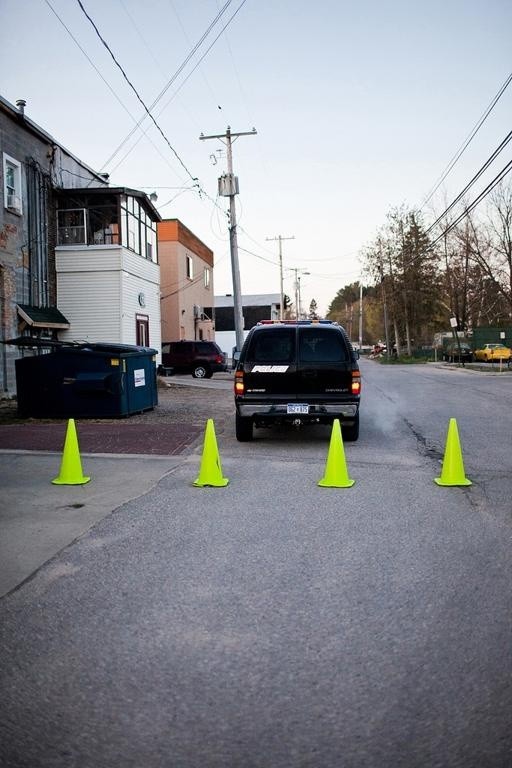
[0,336,158,420]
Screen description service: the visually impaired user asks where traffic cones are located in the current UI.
[434,417,473,486]
[51,418,91,485]
[191,417,229,487]
[317,417,355,488]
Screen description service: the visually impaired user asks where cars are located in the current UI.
[474,343,512,362]
[442,342,474,362]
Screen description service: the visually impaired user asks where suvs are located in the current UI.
[162,339,229,379]
[233,320,361,441]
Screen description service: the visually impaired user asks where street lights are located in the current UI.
[297,271,311,318]
[290,268,299,319]
